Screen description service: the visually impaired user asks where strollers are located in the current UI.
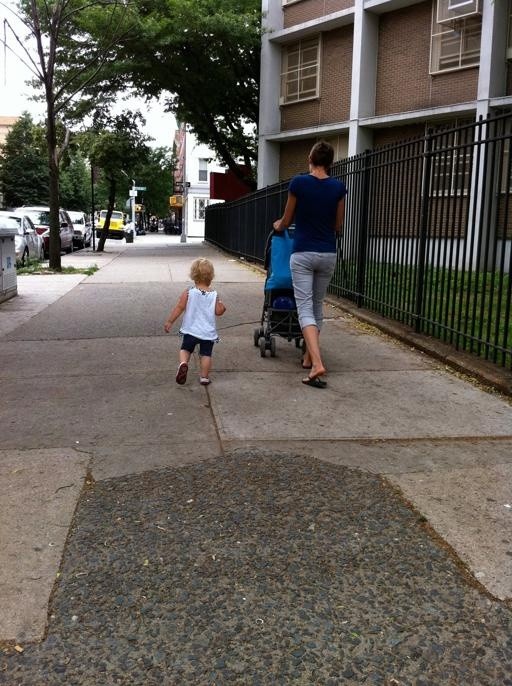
[255,225,310,355]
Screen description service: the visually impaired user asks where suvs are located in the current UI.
[95,209,127,239]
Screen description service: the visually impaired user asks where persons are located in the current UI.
[163,257,227,386]
[160,218,182,236]
[271,138,348,390]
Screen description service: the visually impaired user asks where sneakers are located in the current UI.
[175,362,189,385]
[199,377,210,385]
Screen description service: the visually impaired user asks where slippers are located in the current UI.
[302,375,328,389]
[301,358,312,369]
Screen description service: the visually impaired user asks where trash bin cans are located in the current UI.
[0,228,19,305]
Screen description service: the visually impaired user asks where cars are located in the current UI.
[0,205,92,268]
